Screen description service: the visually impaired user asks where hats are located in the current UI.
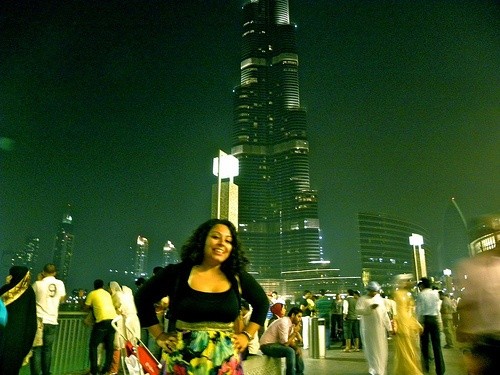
[367,281,380,291]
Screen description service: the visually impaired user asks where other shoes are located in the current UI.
[86,371,97,375]
[352,348,360,352]
[343,349,352,353]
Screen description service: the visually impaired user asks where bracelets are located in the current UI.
[240,331,254,342]
[155,332,164,343]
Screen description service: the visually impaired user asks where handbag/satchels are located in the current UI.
[32,316,44,346]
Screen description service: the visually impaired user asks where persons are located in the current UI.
[438,290,465,349]
[390,273,425,375]
[0,263,67,375]
[267,303,303,342]
[355,282,392,375]
[259,307,305,375]
[455,256,500,375]
[271,291,286,304]
[133,219,269,375]
[135,264,175,289]
[311,289,361,352]
[416,277,446,375]
[82,279,141,375]
[300,290,314,349]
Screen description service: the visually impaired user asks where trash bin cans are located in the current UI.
[306,316,327,359]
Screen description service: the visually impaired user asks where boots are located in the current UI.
[107,350,121,375]
